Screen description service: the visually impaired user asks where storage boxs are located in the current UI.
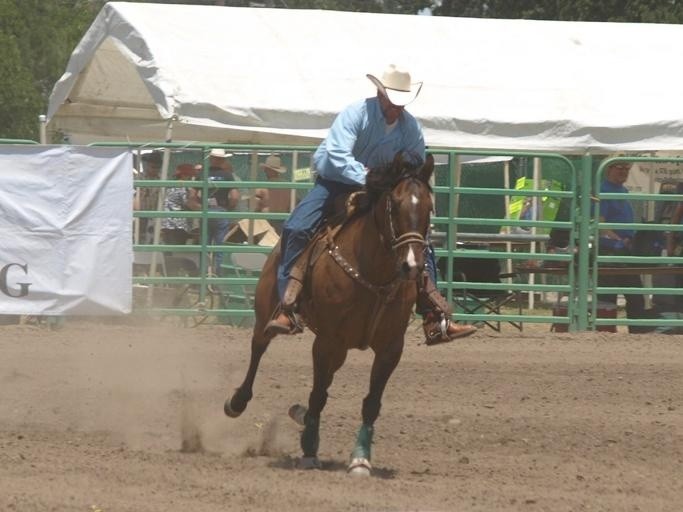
[554,301,616,332]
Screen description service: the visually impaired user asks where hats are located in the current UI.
[259,155,287,173]
[366,64,423,106]
[173,149,232,174]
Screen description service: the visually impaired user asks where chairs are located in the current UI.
[231,252,269,328]
[437,246,523,333]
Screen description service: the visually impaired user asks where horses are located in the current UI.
[223,147,435,481]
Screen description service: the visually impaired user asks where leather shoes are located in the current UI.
[264,310,303,334]
[423,310,477,345]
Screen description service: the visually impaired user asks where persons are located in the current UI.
[133,152,162,276]
[260,64,480,344]
[159,161,202,289]
[590,158,669,338]
[201,149,242,281]
[248,153,303,242]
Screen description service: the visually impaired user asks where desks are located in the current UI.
[516,266,683,334]
